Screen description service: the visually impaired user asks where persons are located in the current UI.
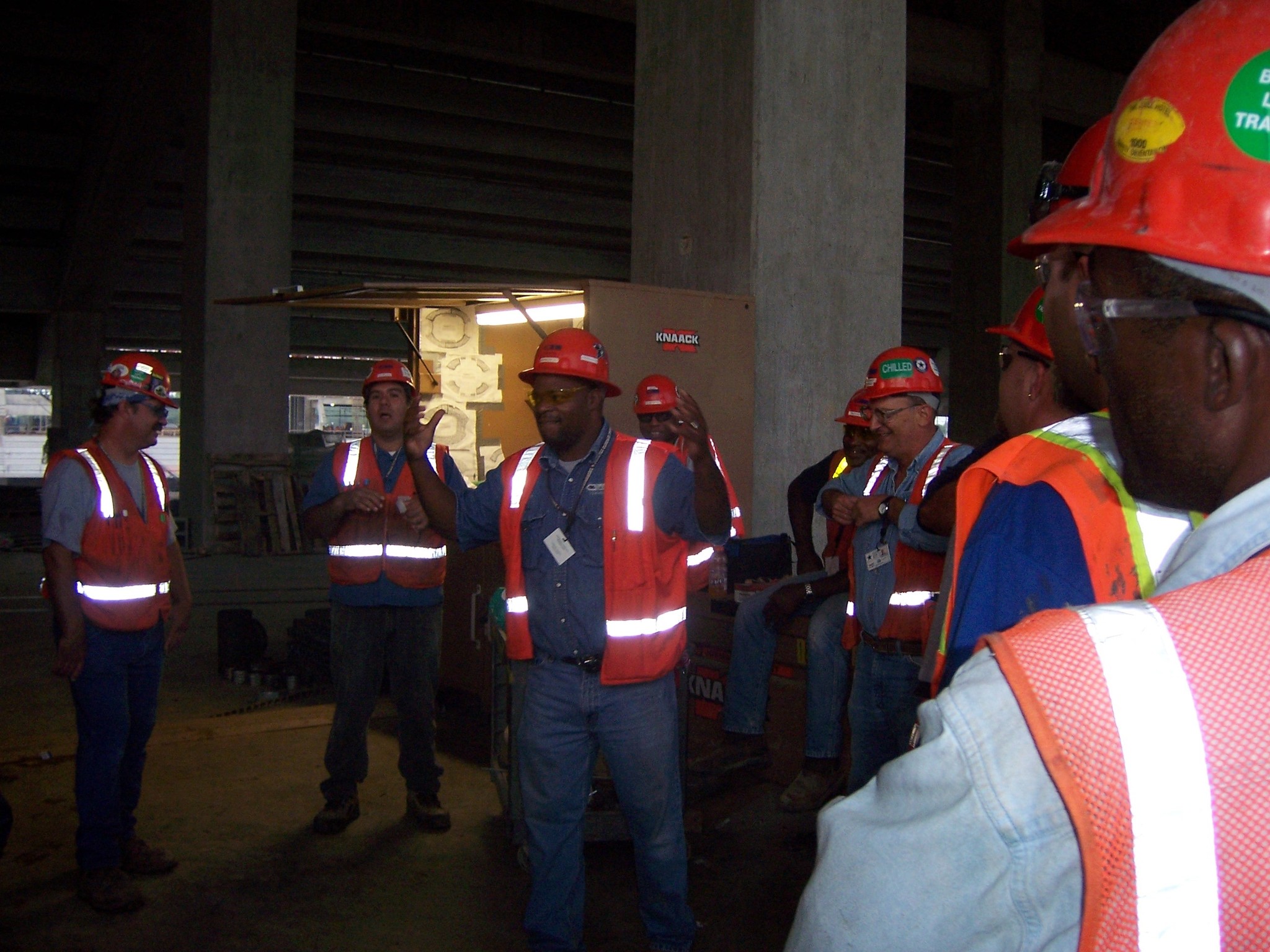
[297,360,470,835]
[41,353,195,914]
[634,112,1222,829]
[788,1,1270,952]
[399,327,730,952]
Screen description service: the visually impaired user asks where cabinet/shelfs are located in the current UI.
[438,538,505,703]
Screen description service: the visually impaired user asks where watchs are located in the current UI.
[877,495,895,520]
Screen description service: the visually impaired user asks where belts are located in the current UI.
[563,654,603,673]
[863,632,922,657]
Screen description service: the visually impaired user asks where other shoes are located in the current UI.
[407,789,450,827]
[84,868,142,911]
[313,796,361,831]
[127,835,177,871]
[781,772,831,810]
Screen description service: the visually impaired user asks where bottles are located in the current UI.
[707,546,728,596]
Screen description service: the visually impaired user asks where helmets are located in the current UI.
[859,347,943,399]
[1020,0,1269,276]
[362,358,417,397]
[1008,112,1112,258]
[633,375,679,414]
[100,352,180,409]
[986,284,1056,359]
[834,388,873,428]
[518,327,621,397]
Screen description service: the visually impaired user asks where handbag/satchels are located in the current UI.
[724,533,796,595]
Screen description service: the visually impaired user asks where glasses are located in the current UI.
[1074,280,1269,357]
[1000,345,1050,371]
[1035,247,1076,284]
[860,404,921,422]
[1028,159,1090,222]
[141,401,170,417]
[527,385,587,407]
[637,411,673,423]
